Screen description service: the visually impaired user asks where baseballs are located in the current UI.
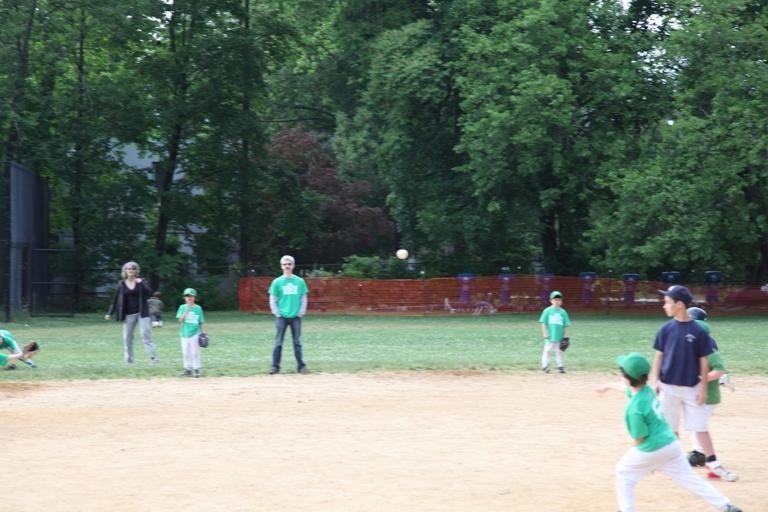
[396,248,409,261]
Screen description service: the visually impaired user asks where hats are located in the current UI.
[549,290,561,299]
[617,353,651,380]
[659,285,691,303]
[688,307,710,334]
[181,288,196,297]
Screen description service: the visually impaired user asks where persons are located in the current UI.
[176,288,208,378]
[687,320,727,467]
[1,351,22,365]
[688,307,708,321]
[105,262,157,363]
[596,352,744,512]
[652,286,739,482]
[0,329,37,368]
[147,291,165,327]
[268,255,311,374]
[538,290,570,373]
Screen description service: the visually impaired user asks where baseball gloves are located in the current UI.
[199,333,209,348]
[560,336,570,350]
[23,341,39,359]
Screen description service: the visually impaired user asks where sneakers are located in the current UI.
[544,366,566,373]
[707,466,736,481]
[269,368,278,373]
[180,369,191,376]
[299,368,308,374]
[195,370,199,376]
[688,451,705,467]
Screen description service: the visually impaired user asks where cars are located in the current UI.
[705,284,768,305]
